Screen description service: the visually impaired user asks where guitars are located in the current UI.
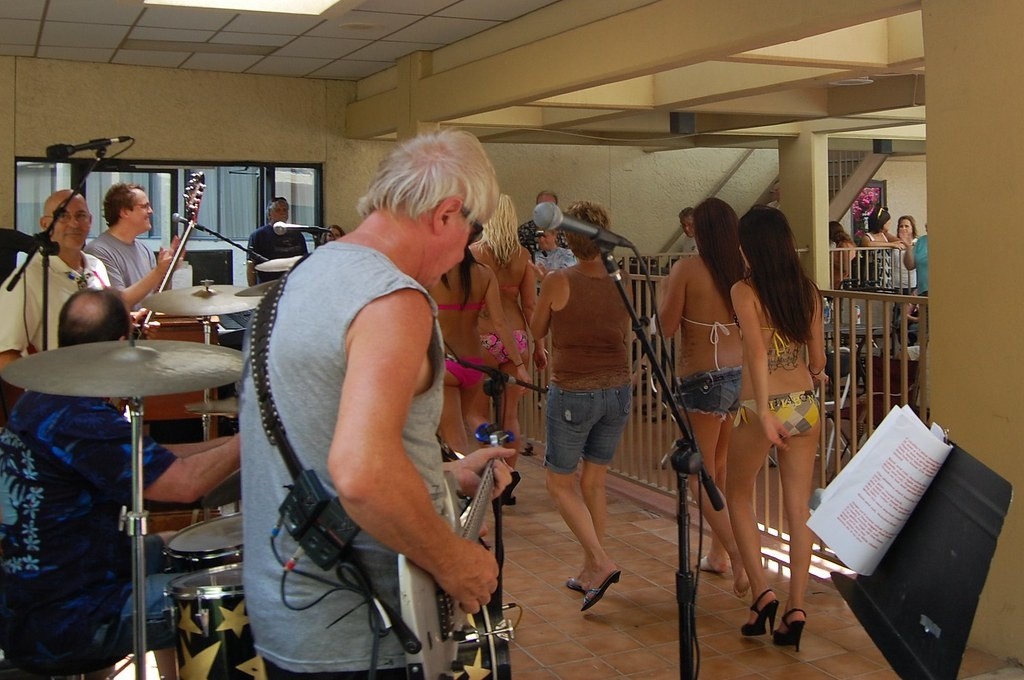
[132,169,207,339]
[398,422,517,679]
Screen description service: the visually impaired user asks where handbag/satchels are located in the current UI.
[851,234,882,286]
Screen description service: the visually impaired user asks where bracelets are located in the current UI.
[809,364,823,375]
[515,362,525,368]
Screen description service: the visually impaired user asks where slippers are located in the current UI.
[698,556,727,574]
[580,570,621,612]
[567,573,587,594]
[492,471,521,506]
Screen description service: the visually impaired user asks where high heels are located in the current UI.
[774,608,806,652]
[741,590,779,636]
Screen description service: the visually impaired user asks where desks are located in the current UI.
[0,318,222,532]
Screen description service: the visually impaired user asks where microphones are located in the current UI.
[171,213,205,231]
[273,221,331,235]
[46,135,130,160]
[532,203,634,247]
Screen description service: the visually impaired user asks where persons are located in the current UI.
[528,199,639,612]
[0,288,242,680]
[238,128,517,680]
[829,208,930,425]
[731,203,827,653]
[666,207,700,261]
[419,193,550,506]
[84,181,186,319]
[0,189,162,425]
[518,190,581,276]
[659,198,752,599]
[246,196,309,288]
[767,180,779,212]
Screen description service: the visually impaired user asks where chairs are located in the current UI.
[645,345,921,476]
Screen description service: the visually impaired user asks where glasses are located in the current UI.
[44,211,92,224]
[272,197,286,202]
[877,208,885,220]
[435,198,483,246]
[130,203,151,212]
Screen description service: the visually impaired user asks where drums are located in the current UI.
[163,563,267,680]
[164,511,245,571]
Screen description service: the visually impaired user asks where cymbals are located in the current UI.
[233,279,278,298]
[255,253,302,273]
[139,279,265,316]
[183,398,238,415]
[1,338,244,400]
